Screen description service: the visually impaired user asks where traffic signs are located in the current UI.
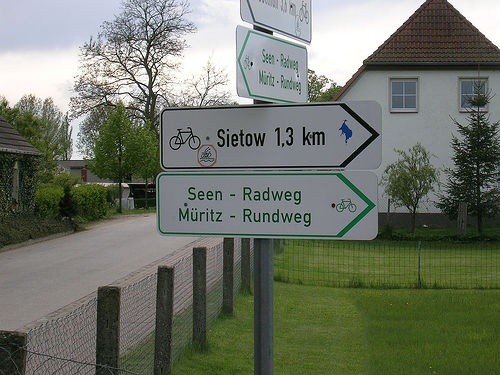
[155,170,379,241]
[159,100,382,173]
[239,0,314,44]
[230,25,309,107]
